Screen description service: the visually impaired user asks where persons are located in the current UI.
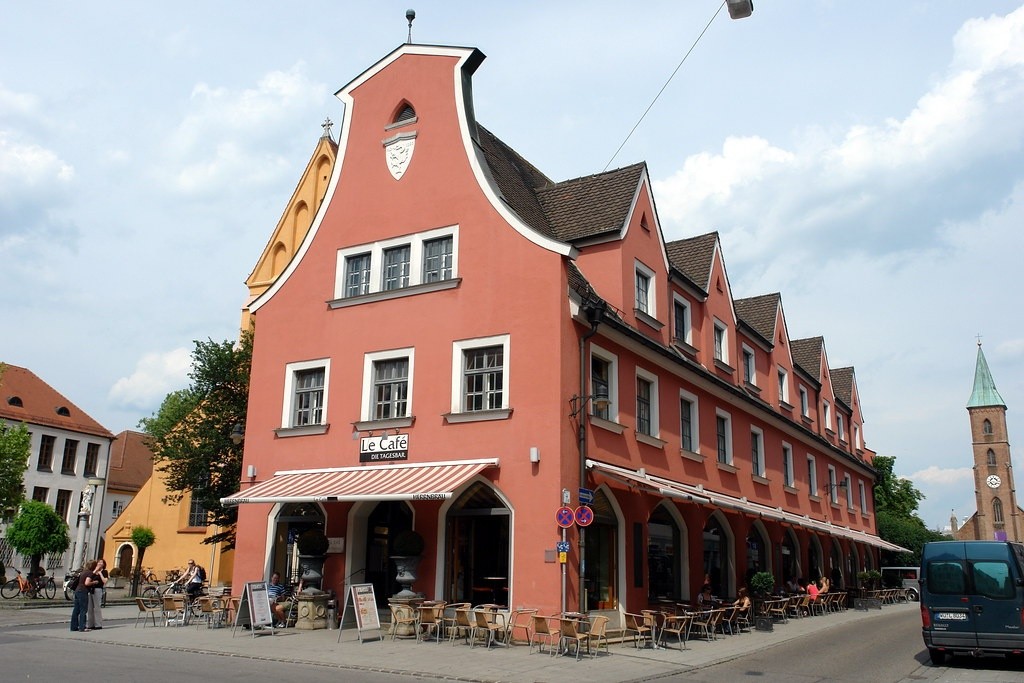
[779,577,806,598]
[730,586,751,623]
[806,580,820,601]
[267,571,287,601]
[272,577,305,628]
[697,584,719,611]
[818,577,831,594]
[70,559,108,632]
[174,560,202,605]
[754,586,773,604]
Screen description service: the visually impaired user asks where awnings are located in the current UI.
[220,458,500,506]
[584,458,914,555]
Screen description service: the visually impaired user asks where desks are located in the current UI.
[563,613,590,653]
[149,598,184,626]
[483,606,510,646]
[198,595,239,629]
[415,602,437,634]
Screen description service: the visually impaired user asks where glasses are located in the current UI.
[188,563,193,565]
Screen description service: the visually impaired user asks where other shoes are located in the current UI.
[88,626,102,629]
[83,629,90,632]
[279,623,286,627]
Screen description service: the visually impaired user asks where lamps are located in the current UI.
[248,464,257,478]
[824,481,847,496]
[726,0,753,20]
[381,428,388,440]
[529,446,541,463]
[229,424,245,444]
[569,384,611,419]
[352,430,360,440]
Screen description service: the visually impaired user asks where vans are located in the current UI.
[881,567,920,601]
[917,540,1024,664]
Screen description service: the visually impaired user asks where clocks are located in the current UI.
[986,474,1002,488]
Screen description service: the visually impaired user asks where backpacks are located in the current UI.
[194,565,206,580]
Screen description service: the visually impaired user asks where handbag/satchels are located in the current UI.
[67,575,81,591]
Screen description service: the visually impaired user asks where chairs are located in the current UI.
[387,600,615,662]
[752,591,847,625]
[272,594,300,628]
[135,594,240,631]
[621,603,751,652]
[869,588,909,605]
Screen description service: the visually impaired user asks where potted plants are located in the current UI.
[750,571,778,630]
[857,569,885,591]
[388,530,430,596]
[106,568,126,589]
[296,528,330,596]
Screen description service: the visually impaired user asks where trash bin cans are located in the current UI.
[327,600,337,629]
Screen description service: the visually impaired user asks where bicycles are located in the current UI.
[139,567,157,585]
[64,566,84,600]
[33,572,57,600]
[130,566,145,584]
[1,566,39,600]
[162,582,183,598]
[163,585,209,619]
[141,580,169,608]
[165,571,175,584]
[172,570,181,582]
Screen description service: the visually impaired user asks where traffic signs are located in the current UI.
[578,487,595,505]
